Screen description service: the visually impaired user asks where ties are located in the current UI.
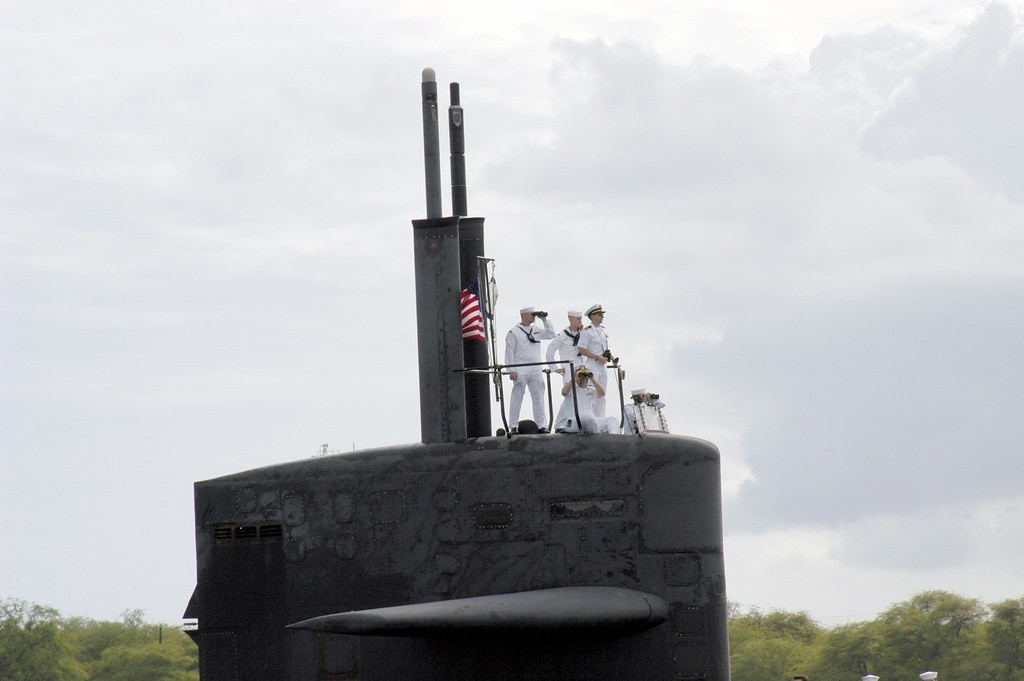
[564,329,581,346]
[520,326,540,343]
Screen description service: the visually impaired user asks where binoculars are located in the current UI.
[602,350,611,362]
[576,369,593,378]
[649,394,659,399]
[532,311,548,316]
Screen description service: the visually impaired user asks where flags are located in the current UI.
[461,278,487,342]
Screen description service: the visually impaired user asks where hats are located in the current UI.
[520,307,535,314]
[568,311,582,317]
[630,387,645,395]
[574,363,591,373]
[584,304,606,316]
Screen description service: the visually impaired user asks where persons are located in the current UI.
[504,307,557,434]
[547,304,620,434]
[624,388,665,435]
